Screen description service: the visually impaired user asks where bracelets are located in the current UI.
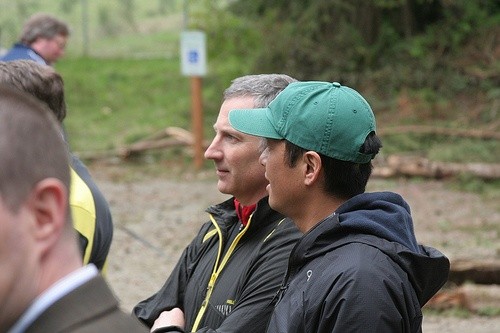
[152,325,185,333]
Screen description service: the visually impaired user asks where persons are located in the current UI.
[0,13,151,333]
[131,73,301,333]
[229,82,451,333]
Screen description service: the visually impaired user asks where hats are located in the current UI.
[228,81,377,163]
